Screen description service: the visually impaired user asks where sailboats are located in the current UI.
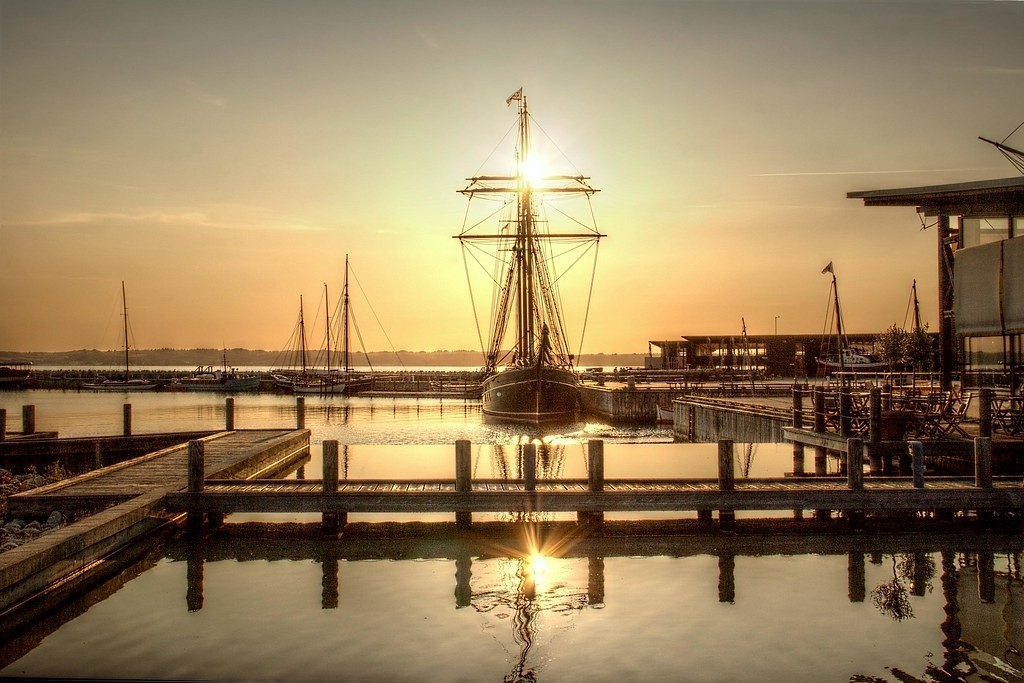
[449,83,609,422]
[261,253,487,393]
[78,280,160,391]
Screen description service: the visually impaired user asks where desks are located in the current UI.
[885,398,928,435]
[990,396,1024,435]
[850,392,891,419]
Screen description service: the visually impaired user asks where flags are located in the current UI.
[822,262,835,275]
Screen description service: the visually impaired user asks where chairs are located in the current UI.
[810,385,1024,440]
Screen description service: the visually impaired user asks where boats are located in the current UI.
[0,359,34,388]
[164,341,262,391]
